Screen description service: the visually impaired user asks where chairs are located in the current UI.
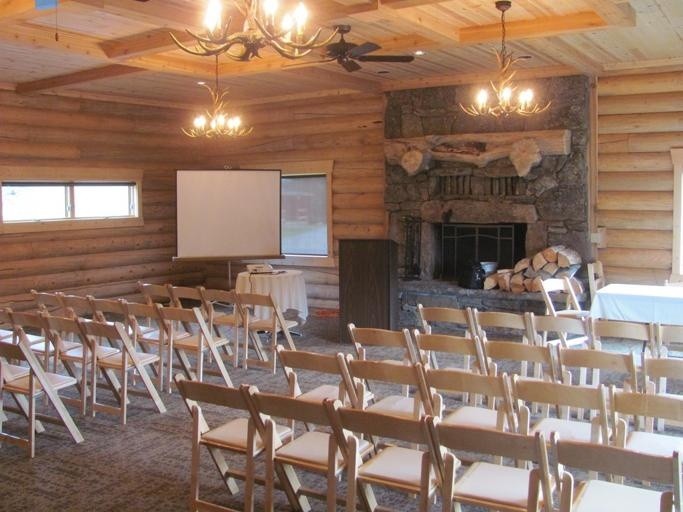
[586,261,606,305]
[536,274,597,350]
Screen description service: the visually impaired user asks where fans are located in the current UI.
[317,22,415,73]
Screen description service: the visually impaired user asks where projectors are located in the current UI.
[245,264,273,274]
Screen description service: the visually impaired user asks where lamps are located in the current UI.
[172,36,259,140]
[166,0,342,64]
[456,2,555,119]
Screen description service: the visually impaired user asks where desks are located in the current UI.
[588,282,683,348]
[231,267,309,340]
